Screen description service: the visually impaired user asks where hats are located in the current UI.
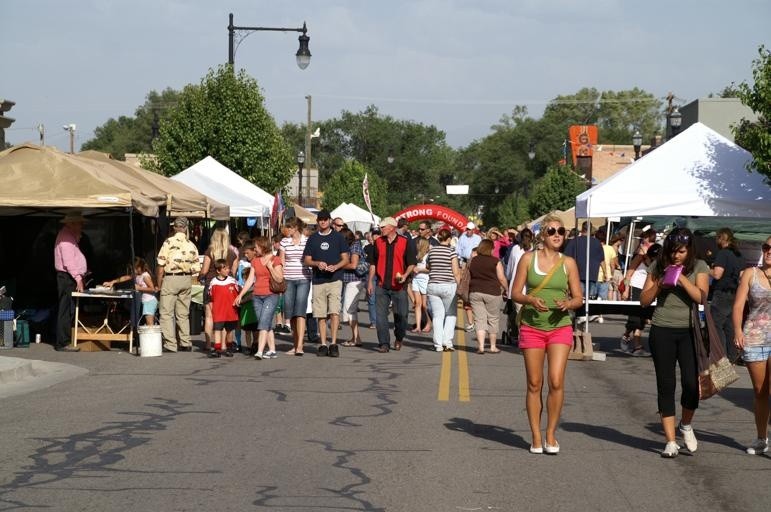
[466,221,474,230]
[317,208,331,219]
[377,216,398,228]
[169,218,191,226]
[58,212,87,223]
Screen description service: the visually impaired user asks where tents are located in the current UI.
[169,154,278,241]
[0,143,167,356]
[328,202,380,238]
[576,124,771,335]
[169,171,270,243]
[143,166,232,253]
[288,200,318,226]
[68,151,209,273]
[560,205,606,239]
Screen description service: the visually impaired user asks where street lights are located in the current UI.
[62,125,73,153]
[227,13,312,70]
[297,151,305,206]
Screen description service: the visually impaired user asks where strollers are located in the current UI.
[501,285,520,346]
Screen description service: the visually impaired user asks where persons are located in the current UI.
[412,221,439,330]
[196,228,239,354]
[456,221,482,274]
[208,259,238,358]
[238,240,255,288]
[427,229,461,353]
[732,236,771,456]
[303,209,349,357]
[238,231,251,253]
[505,228,537,335]
[303,222,329,344]
[354,231,372,258]
[368,217,417,353]
[499,227,520,276]
[55,213,87,352]
[239,267,259,355]
[487,228,510,259]
[561,227,579,254]
[640,228,709,458]
[398,218,458,247]
[707,227,746,364]
[407,281,417,309]
[411,237,433,333]
[564,221,608,297]
[280,216,312,356]
[156,217,201,353]
[462,247,478,332]
[273,233,292,336]
[512,213,583,454]
[595,231,617,299]
[364,230,382,329]
[327,217,344,330]
[466,239,508,354]
[339,228,366,347]
[610,229,663,357]
[234,237,283,360]
[103,256,158,325]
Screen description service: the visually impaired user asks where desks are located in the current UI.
[572,300,668,352]
[73,288,137,353]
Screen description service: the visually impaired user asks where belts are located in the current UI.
[164,272,194,277]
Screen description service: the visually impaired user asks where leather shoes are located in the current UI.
[545,438,560,455]
[178,345,193,353]
[394,340,401,351]
[56,343,80,353]
[162,347,174,353]
[528,442,543,454]
[377,347,389,353]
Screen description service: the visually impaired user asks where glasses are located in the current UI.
[333,222,344,227]
[418,227,428,232]
[762,242,771,253]
[539,226,567,237]
[667,233,692,244]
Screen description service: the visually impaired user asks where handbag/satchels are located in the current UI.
[267,256,288,292]
[686,285,741,401]
[349,242,370,278]
[515,255,566,326]
[455,259,472,304]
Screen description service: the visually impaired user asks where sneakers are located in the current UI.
[588,316,599,322]
[576,316,586,325]
[678,423,698,454]
[593,317,605,323]
[206,351,221,358]
[254,352,263,359]
[746,438,770,455]
[225,349,234,357]
[329,344,340,357]
[316,345,328,357]
[630,347,650,357]
[431,345,444,351]
[262,351,278,359]
[620,334,631,352]
[444,345,455,352]
[464,323,475,332]
[661,439,679,458]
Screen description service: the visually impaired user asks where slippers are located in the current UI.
[487,347,501,354]
[285,348,295,355]
[341,340,363,347]
[474,349,485,353]
[296,349,306,355]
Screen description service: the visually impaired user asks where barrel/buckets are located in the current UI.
[136,325,163,357]
